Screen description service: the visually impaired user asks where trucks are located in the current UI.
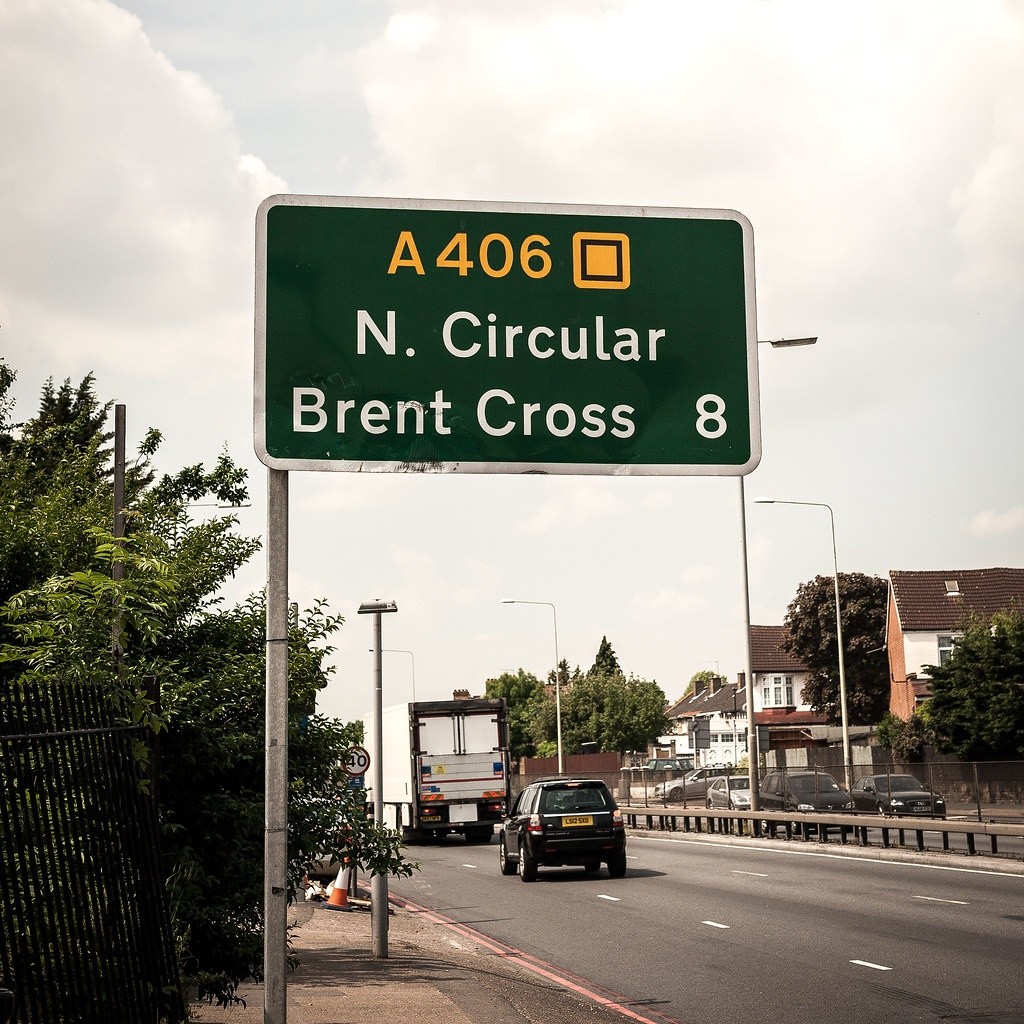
[361,688,509,847]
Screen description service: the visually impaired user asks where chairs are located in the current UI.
[573,791,588,801]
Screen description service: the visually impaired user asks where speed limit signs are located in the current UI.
[341,745,370,776]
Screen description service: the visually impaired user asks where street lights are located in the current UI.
[738,333,823,832]
[369,649,415,703]
[356,598,400,958]
[753,497,852,792]
[500,600,563,776]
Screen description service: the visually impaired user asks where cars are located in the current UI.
[636,757,703,781]
[705,775,764,811]
[654,765,741,803]
[849,774,947,820]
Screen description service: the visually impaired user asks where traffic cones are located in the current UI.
[322,854,354,912]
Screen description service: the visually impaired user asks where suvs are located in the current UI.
[499,776,627,883]
[757,770,859,835]
